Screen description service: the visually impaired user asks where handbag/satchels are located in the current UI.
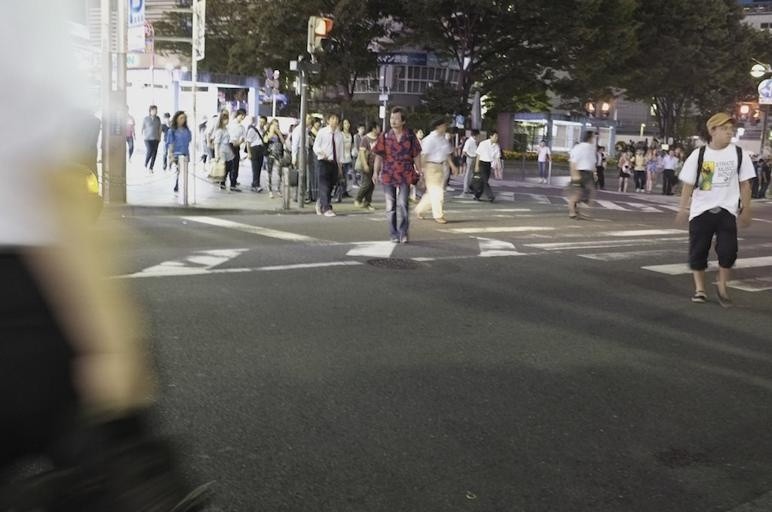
[469,175,484,197]
[355,149,370,172]
[334,183,342,202]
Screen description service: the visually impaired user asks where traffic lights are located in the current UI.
[600,102,610,119]
[585,101,596,119]
[307,15,336,57]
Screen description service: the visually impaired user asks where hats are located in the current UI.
[706,113,738,132]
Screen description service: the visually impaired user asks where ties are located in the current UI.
[332,133,336,162]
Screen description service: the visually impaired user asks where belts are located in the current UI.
[425,159,445,164]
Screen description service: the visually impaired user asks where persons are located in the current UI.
[459,129,503,202]
[126,105,137,163]
[371,106,424,245]
[199,107,285,199]
[284,113,459,224]
[676,113,757,307]
[536,131,768,221]
[141,105,192,197]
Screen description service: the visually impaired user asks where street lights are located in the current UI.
[747,58,771,79]
[739,101,767,158]
[267,68,281,117]
[531,124,546,152]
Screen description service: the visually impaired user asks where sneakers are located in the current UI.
[354,200,364,209]
[391,235,408,243]
[416,212,423,220]
[435,217,447,223]
[315,203,321,215]
[221,181,283,197]
[366,204,376,211]
[324,210,336,217]
[538,178,546,183]
[715,290,733,308]
[691,290,707,303]
